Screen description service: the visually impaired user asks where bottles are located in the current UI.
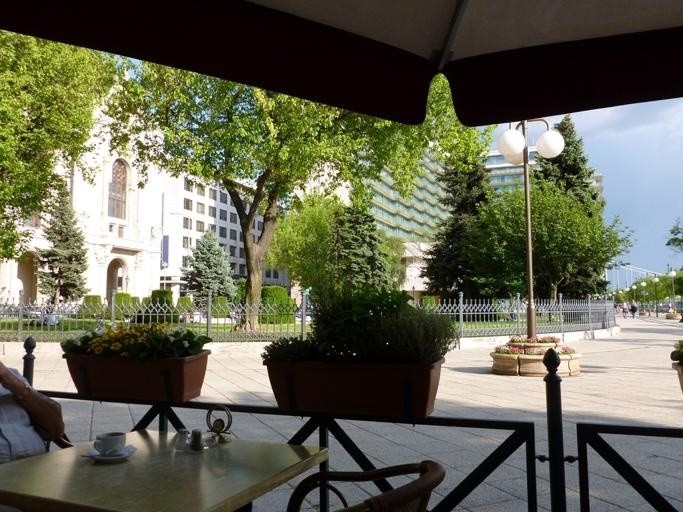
[189,429,204,451]
[176,429,187,450]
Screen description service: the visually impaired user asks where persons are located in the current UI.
[614,299,640,319]
[0,359,66,443]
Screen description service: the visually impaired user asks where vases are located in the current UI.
[63,350,212,403]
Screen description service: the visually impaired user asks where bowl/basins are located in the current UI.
[188,432,219,449]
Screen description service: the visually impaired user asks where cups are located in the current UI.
[93,432,127,454]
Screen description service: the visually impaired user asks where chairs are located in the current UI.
[46,436,74,453]
[288,460,445,512]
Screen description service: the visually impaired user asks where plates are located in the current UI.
[81,445,137,462]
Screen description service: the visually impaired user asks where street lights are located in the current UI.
[668,271,675,314]
[612,282,646,311]
[651,277,658,317]
[497,117,565,341]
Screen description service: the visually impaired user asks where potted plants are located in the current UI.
[670,340,683,394]
[261,284,461,418]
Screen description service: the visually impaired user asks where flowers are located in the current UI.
[496,336,574,354]
[61,320,213,361]
